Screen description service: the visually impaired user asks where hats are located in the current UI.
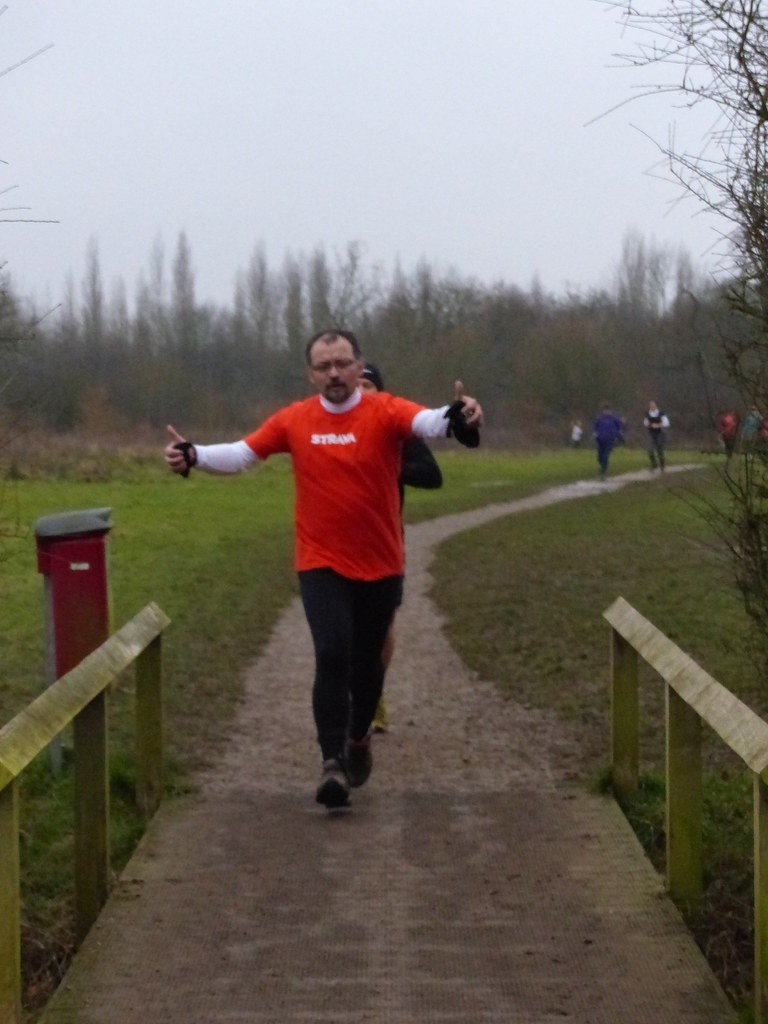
[359,362,384,392]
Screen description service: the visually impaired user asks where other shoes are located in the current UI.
[370,697,390,737]
[314,758,354,813]
[344,727,372,787]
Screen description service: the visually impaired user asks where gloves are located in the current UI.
[443,400,479,449]
[170,441,193,480]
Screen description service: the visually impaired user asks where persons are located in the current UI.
[571,421,583,448]
[742,407,768,459]
[166,329,485,807]
[357,363,443,732]
[721,410,740,460]
[591,405,628,483]
[644,401,671,473]
